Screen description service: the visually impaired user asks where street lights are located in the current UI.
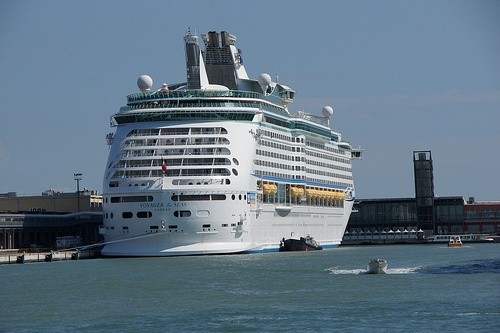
[74,173,82,219]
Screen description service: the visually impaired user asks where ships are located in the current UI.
[100,24,362,257]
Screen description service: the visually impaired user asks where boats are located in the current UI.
[284,236,322,251]
[366,258,388,273]
[448,236,462,248]
[427,235,478,243]
